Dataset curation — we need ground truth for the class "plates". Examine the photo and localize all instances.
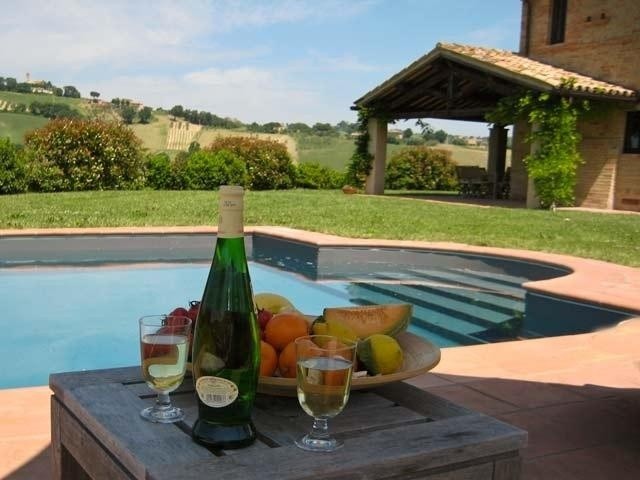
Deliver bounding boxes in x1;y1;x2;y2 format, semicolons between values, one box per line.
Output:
184;329;441;398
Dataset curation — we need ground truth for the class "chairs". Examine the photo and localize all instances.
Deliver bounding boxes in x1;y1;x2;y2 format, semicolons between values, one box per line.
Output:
454;165;512;201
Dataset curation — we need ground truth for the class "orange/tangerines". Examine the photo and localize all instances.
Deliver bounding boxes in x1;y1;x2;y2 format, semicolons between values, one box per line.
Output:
259;314;319;377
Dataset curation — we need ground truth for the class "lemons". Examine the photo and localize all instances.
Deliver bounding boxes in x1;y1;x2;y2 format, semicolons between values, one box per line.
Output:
362;333;404;374
312;320;359;350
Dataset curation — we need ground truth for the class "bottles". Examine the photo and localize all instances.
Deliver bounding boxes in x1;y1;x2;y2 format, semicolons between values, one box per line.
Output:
189;186;261;449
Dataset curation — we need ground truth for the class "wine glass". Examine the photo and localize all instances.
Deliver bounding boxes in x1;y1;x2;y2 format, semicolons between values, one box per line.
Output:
293;335;357;453
139;315;194;424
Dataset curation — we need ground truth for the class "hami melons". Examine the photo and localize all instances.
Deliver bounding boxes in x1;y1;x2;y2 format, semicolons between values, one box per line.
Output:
323;304;413;340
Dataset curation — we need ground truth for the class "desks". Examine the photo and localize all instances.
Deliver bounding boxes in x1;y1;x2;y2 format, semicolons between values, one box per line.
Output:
47;364;530;480
